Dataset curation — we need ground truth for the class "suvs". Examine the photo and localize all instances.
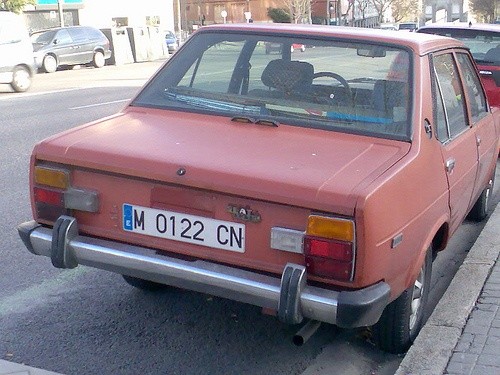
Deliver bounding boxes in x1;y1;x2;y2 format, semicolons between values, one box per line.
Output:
29;25;112;73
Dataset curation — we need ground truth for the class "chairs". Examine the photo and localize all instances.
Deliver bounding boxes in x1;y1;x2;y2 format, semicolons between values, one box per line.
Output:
247;59;314;101
370;78;409;113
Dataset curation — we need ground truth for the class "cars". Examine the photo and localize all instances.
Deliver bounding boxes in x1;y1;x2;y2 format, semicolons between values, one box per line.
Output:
164;30;176;54
1;11;35;93
387;22;500;107
16;22;500;355
382;23;416;32
